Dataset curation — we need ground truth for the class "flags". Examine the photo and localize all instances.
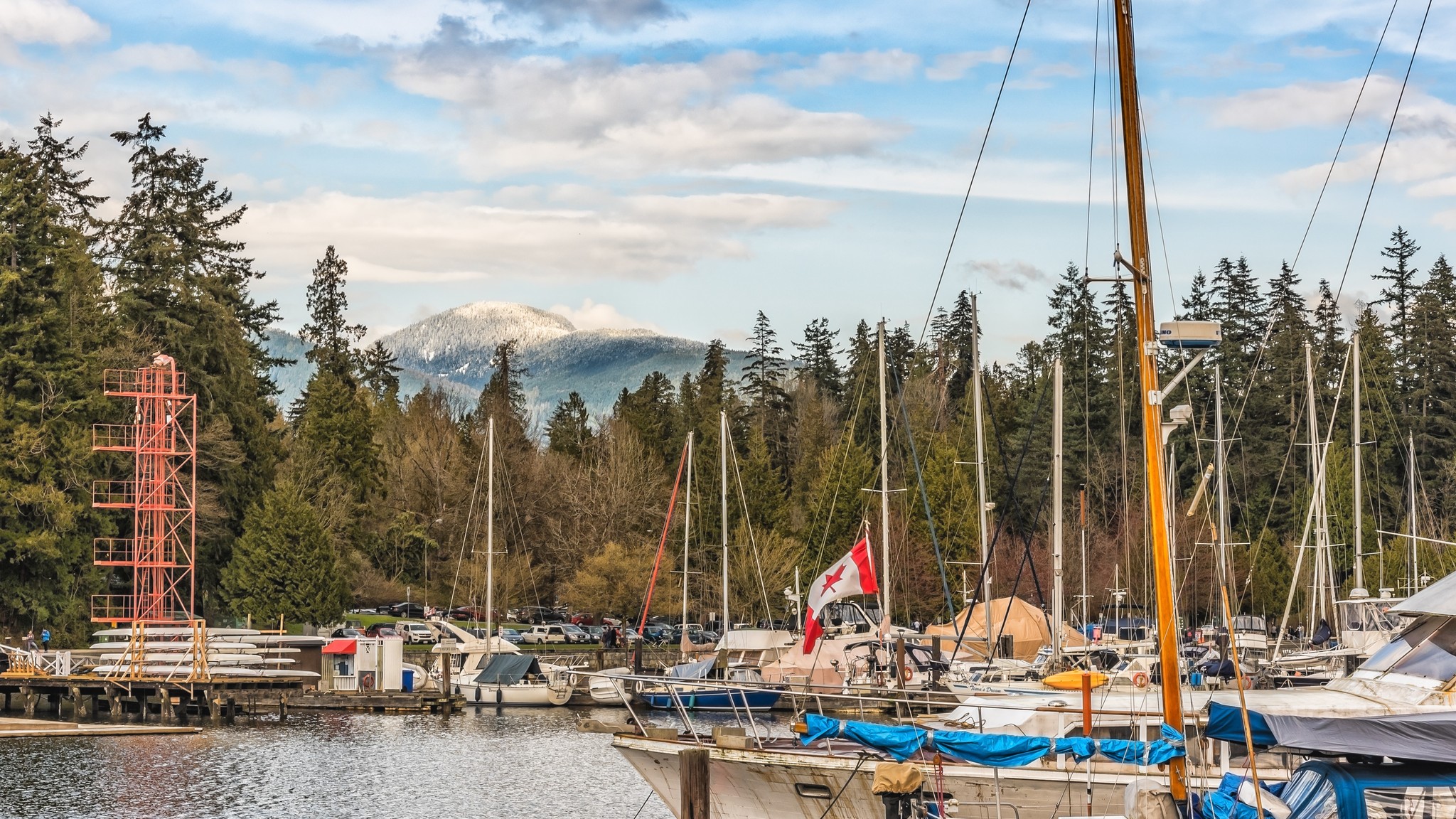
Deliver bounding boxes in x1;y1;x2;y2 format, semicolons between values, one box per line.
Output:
426;608;435;615
803;527;879;657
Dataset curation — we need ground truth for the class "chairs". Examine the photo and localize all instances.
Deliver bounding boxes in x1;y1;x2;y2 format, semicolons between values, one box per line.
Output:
688;631;692;634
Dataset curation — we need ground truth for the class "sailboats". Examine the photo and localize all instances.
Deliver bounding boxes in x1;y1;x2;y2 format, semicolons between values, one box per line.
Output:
402;0;1456;819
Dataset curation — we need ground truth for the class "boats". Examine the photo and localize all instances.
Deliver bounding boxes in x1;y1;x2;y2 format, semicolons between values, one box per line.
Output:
89;627;321;677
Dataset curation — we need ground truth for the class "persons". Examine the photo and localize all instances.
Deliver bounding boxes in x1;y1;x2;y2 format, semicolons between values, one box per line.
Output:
40;627;50;653
27;631;40;653
601;626;623;649
1271;623;1304;641
911;620;923;634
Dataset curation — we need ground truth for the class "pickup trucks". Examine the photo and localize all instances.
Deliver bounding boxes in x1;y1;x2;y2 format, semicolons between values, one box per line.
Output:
520;625;566;645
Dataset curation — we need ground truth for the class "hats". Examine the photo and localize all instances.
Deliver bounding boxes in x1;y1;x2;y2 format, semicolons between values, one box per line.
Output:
617;627;620;630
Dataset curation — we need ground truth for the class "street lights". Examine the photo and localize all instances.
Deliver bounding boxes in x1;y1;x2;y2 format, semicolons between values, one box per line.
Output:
425;519;442;621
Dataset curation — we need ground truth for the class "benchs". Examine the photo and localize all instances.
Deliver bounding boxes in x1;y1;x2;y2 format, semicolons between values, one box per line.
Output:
599;640;632;648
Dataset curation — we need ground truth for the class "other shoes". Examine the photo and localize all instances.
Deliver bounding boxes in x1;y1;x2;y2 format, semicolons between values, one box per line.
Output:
44;651;48;653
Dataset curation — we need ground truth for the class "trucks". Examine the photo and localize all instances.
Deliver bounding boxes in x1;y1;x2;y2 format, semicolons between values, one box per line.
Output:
394;620;436;645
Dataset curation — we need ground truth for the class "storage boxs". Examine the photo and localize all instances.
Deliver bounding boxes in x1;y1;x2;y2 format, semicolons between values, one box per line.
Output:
711;726;746;742
717;735;754;749
644;728;679;742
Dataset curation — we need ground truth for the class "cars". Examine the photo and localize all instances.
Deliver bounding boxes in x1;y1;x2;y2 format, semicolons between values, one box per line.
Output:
368;628;404;645
1200;625;1218;638
570;613;613;627
493;628;524;644
603;613;630;629
670;628;703;645
699;631;721;644
451;606;502;623
614;628;644;644
388;602;435;619
331;628;366;638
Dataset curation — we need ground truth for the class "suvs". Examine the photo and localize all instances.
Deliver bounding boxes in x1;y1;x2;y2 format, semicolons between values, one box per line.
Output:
516;606;565;625
757;618;796;630
551;622;591;644
578;625;606;644
635;626;674;645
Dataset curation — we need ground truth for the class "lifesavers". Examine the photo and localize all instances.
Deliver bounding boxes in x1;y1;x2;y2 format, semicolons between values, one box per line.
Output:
437;674;442;678
363;675;374;688
1133;672;1148;688
1241;676;1251;690
636;681;642;694
570;673;577;686
904;667;913;681
